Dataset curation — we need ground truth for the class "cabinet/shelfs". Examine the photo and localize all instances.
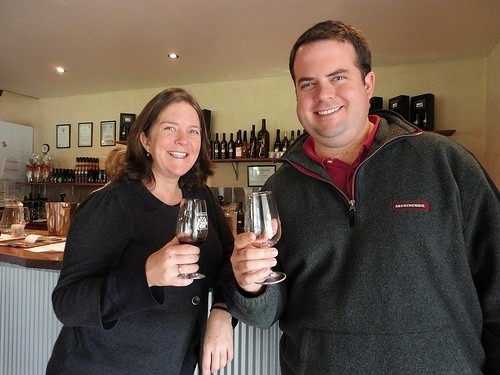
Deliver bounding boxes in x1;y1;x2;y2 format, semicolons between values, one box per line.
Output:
16;181;105;231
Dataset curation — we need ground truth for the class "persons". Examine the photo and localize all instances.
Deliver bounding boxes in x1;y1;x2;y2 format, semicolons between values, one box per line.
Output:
46;87;238;375
227;21;500;375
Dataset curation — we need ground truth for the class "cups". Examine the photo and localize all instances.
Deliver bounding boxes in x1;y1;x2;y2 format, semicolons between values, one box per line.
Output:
99;170;110;183
11;224;24;238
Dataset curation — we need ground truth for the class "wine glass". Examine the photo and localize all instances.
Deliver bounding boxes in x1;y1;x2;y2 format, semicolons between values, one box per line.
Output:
175;198;208;280
243;191;287;285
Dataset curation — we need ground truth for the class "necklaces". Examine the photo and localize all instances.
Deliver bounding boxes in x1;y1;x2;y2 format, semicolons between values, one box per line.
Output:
159;188;180;205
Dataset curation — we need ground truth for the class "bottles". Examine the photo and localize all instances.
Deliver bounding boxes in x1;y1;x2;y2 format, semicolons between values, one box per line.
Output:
50;156;100;183
208;119;307;160
237;202;244;234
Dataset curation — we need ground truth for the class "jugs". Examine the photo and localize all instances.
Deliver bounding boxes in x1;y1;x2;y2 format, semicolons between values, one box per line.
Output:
0;197;30;234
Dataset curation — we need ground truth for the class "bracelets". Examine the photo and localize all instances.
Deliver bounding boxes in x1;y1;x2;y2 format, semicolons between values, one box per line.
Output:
210;305;227;311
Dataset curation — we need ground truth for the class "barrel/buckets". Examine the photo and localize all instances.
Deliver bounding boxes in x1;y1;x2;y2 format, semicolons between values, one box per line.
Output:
45;201;77;237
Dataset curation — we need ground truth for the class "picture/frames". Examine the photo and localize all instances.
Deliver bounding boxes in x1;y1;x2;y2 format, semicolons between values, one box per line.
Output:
78;122;93;147
56;124;71;148
100;121;116;146
247;165;277;188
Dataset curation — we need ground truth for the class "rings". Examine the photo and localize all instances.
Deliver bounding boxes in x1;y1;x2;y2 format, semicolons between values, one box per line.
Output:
178;265;182;274
